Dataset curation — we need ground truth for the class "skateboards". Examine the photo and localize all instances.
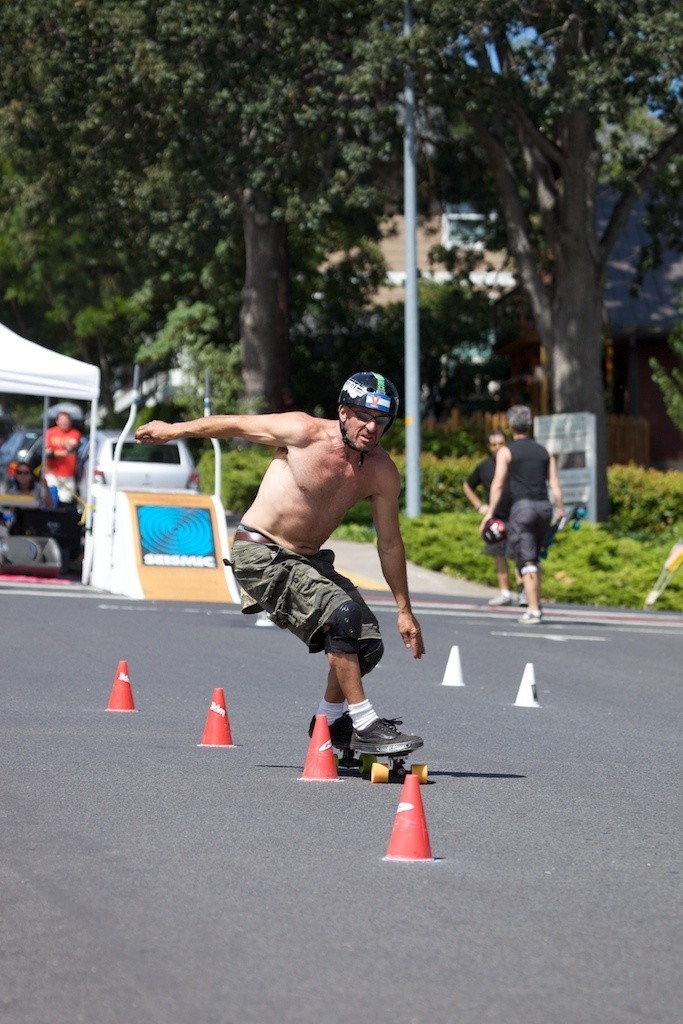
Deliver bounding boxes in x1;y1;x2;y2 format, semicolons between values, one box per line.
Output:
537;501;588;561
328;735;429;784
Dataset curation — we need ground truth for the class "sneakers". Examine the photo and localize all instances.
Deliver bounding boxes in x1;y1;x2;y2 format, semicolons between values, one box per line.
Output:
307;710;353;751
350;716;424;753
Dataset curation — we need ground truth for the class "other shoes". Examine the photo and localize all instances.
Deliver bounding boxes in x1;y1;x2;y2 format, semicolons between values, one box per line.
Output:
488;592;512;606
518;611;543;624
518;589;528;607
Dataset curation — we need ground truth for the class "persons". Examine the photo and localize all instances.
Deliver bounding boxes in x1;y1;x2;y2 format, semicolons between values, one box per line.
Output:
478;406;564;623
7;462;53;536
463;429;529;606
136;371;426;753
48;411;82;505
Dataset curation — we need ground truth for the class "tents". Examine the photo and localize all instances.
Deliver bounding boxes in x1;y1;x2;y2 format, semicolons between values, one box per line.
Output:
1;323;101;582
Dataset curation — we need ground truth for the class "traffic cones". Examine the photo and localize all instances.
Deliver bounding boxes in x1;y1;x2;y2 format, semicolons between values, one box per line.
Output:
512;663;540;708
198;688;237;747
439;646;467;686
297;713;342;781
104;660;138;713
381;775;435;861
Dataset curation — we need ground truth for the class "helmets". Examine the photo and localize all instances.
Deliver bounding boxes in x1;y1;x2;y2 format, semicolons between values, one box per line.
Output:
483;518;507;544
338;371;399;417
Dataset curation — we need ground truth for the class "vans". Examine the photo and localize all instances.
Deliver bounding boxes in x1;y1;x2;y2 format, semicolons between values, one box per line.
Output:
78;430;198;524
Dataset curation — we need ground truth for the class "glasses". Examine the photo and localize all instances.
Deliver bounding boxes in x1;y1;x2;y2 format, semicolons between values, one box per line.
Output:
344;404;389;425
16;470;31;474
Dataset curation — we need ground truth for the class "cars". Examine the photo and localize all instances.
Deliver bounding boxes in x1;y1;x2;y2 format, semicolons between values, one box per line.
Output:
0;431;43;493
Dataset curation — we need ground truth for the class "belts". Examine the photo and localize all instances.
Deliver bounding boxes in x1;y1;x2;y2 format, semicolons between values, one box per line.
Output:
235;532;274;544
